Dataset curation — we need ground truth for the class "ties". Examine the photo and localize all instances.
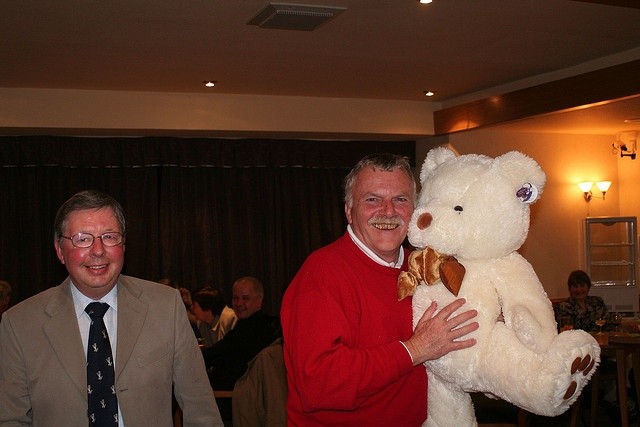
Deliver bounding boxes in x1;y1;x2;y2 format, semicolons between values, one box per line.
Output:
84;302;119;427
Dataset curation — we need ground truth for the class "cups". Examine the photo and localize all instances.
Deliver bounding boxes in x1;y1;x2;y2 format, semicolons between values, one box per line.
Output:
559;316;574;332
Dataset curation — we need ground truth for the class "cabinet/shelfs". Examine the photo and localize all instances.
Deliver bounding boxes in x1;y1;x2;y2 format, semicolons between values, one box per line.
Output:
580;216;639;317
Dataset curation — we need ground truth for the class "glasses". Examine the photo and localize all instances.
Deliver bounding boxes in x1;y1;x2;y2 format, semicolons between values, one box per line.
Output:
62;233;125;247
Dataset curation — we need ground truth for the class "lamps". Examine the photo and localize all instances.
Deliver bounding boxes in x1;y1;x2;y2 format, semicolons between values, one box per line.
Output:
579;180;593;202
597;180;612;200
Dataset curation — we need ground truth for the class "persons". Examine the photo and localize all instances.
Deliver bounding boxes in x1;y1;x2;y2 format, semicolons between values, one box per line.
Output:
0;189;226;426
0;281;12;313
279;153;480;427
556;270;614;332
189;285;238;348
154;278;194;312
202;275;283;421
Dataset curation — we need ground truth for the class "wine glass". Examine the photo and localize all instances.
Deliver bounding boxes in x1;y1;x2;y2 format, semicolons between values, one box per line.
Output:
594;312;606;335
610;312;622;335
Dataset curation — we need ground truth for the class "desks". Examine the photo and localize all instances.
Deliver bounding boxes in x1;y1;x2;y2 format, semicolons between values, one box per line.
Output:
570;330;640;426
